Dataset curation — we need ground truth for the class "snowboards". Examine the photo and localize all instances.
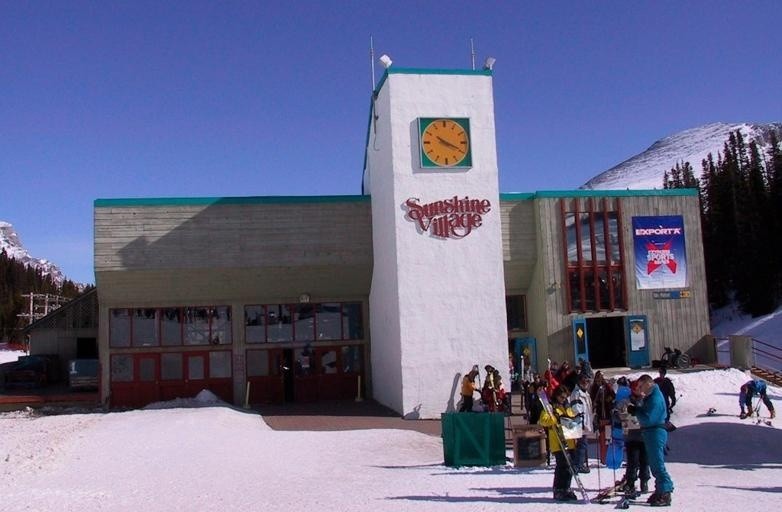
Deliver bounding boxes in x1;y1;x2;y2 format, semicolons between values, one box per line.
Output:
605;385;631;469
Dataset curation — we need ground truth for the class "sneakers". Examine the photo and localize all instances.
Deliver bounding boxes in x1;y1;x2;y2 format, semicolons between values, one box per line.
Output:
553;489;578;502
641;481;648;492
618;479;635;492
646;492;672;507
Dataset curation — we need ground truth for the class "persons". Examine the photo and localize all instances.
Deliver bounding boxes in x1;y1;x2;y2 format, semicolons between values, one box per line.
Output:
459;350;679;507
738;378;775;419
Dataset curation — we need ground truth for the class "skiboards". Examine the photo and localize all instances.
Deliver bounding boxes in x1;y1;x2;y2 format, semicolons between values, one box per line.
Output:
520;355;525;409
595;476;626;503
488;372;499;414
537;386;590;505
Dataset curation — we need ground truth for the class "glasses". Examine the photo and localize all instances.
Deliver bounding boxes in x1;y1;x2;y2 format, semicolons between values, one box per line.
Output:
560;396;569;400
637;379;650;389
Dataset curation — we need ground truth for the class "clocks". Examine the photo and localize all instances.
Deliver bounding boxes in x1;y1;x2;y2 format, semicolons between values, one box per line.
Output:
419;118;473;169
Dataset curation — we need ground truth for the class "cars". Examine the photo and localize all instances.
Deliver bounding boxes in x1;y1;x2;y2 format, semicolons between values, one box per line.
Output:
0;355;68;390
68;359;100;393
